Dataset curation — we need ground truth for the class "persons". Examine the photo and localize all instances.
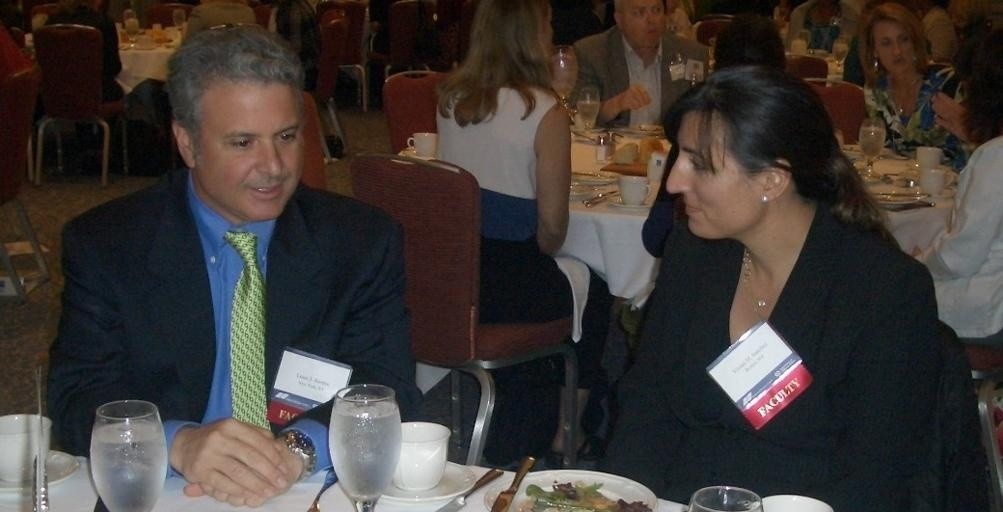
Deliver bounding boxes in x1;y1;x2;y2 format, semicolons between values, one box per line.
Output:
912;7;1003;350
565;0;694;132
772;2;1003;171
186;3;256;38
601;64;942;512
434;2;612;465
2;2;122;173
642;10;787;258
45;21;413;504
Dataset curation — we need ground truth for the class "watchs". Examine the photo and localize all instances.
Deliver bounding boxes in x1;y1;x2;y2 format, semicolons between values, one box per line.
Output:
282;427;317;486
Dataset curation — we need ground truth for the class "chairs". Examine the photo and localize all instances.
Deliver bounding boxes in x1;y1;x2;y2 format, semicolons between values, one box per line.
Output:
961;339;1003;512
351;154;578;469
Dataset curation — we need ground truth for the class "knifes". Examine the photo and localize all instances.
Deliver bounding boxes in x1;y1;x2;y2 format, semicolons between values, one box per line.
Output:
489;456;536;512
435;469;503;512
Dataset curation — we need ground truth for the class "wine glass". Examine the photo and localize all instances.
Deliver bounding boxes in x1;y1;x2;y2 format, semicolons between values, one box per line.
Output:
550;46;579;117
859;117;886;176
689;485;764;512
576;88;600;143
122;8;186;37
790;30;849;75
328;384;403;511
88;399;168;511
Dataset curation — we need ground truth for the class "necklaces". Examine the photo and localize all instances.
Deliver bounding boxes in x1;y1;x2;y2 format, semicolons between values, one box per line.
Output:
743;248;779;325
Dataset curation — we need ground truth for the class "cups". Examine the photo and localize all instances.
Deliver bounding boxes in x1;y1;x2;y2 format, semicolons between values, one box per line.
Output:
393;421;451;492
406;133;436;159
0;413;54;482
762;494;834;512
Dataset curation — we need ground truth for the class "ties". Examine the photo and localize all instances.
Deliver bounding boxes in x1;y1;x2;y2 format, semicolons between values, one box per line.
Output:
224;228;269;430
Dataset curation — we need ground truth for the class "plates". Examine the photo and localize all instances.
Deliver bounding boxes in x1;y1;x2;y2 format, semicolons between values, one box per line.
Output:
0;450;80;491
317;465;532;512
483;469;658;512
381;460;476;501
134;43;160;50
570;123;959;210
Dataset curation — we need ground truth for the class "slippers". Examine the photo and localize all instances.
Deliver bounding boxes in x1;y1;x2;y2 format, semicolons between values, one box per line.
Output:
545;440;602;469
